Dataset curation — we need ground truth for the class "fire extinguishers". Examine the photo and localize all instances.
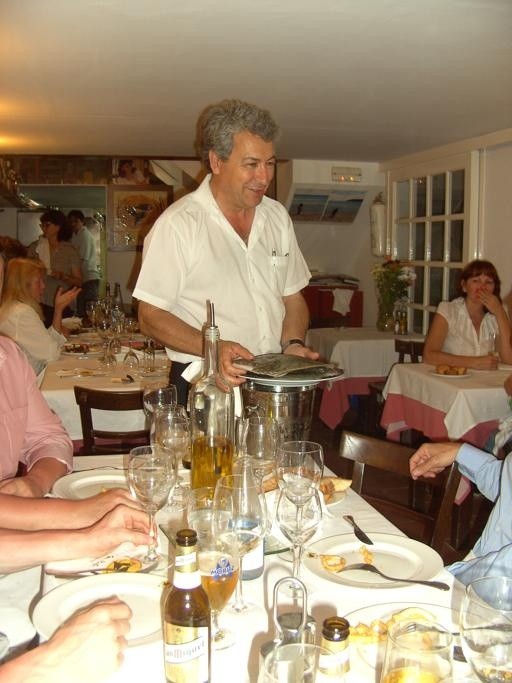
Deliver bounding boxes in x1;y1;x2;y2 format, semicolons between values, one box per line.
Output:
369;191;387;257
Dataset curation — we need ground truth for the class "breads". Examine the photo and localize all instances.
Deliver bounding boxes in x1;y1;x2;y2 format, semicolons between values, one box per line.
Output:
436;364;466;375
258;465;353;504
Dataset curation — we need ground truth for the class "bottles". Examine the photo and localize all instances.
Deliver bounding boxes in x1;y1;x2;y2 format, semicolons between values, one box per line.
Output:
189;300;237;502
102;281;113;305
113;283;124;306
394;309;408;333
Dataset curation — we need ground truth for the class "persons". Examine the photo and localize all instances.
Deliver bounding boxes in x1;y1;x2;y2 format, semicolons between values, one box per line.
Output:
410;441;511;611
68;209;102;318
1;338;75;500
1;256;82;388
1;487;142;530
131;98;320;434
0;506;157;678
422;259;511;370
25;208;83;329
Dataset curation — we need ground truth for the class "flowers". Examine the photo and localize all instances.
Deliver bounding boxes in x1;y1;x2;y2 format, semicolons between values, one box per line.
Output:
369;252;418;314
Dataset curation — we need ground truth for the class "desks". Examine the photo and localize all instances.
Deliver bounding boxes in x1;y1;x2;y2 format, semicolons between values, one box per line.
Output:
300;272;363;325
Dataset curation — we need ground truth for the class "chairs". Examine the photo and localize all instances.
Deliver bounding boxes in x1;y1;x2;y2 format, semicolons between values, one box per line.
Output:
438;451;501;565
363;336;423;440
340;430;457;563
75;384;166;455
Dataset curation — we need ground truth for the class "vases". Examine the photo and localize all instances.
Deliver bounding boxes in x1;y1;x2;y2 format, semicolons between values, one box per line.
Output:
377;299;397;330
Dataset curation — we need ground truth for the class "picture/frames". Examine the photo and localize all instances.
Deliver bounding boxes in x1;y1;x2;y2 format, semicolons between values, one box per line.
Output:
108;185;174;251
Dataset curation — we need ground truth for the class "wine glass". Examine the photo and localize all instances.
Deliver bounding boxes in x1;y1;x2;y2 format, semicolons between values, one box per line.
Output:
128;383;326;613
79;299;158;374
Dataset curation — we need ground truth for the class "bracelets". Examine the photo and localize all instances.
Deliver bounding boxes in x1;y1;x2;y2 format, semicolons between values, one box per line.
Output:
281;338;305;352
64;273;69;282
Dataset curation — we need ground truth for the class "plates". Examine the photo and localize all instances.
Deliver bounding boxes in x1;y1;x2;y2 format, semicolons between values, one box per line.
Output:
237;369;344;387
29;467;511;681
432;372;472;378
61;349;101;355
